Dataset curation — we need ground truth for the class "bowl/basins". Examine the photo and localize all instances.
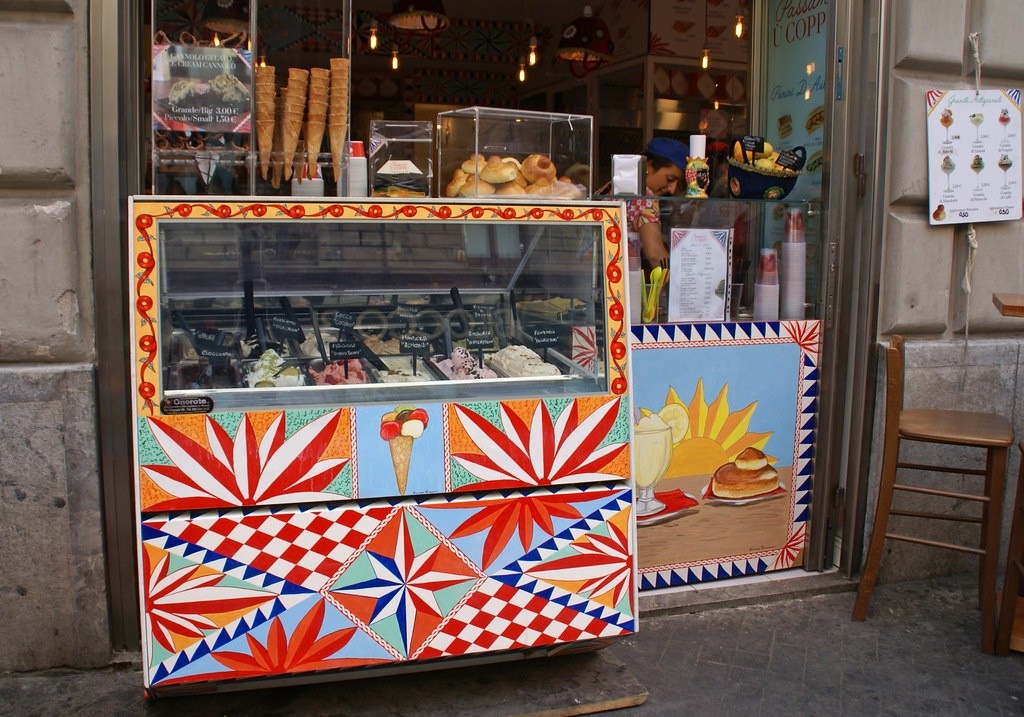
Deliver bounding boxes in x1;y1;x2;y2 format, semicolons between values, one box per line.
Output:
162;321;562;391
290;164;324;196
336;141;368;197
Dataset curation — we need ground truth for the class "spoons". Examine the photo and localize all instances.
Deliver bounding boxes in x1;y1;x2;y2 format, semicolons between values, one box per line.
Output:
641;267;670;323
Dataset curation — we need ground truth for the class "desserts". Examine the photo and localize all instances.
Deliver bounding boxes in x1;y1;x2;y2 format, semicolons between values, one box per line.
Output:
167;74;248;106
941;108;1011;170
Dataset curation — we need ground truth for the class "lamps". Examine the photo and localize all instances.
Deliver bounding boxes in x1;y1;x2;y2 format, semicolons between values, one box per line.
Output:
201;0;252;35
384;0;451;36
555;1;614;77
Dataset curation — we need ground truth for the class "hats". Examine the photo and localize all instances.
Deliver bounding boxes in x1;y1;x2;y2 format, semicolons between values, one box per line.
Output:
648;138;690;171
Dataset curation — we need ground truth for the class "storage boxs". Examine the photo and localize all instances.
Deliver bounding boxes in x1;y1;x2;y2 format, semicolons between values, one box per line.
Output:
539;0;750;77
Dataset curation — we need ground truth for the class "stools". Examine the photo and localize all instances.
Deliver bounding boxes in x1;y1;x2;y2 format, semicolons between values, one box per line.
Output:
852;331;1015;654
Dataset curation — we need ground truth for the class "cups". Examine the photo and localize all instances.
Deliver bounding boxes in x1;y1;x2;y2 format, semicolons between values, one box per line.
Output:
640;284;661;324
753;208;807;322
627;231;642;324
730;283;745;320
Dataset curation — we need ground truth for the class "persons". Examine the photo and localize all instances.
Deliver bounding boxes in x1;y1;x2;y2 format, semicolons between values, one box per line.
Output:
600;137;688;274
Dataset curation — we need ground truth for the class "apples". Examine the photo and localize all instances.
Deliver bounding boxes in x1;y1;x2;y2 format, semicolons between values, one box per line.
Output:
734;140;792;172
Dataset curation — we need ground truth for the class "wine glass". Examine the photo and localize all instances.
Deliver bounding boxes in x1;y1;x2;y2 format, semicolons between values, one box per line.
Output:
940;117;1013;192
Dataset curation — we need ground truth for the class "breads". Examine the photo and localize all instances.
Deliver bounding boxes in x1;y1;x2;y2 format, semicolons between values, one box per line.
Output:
446;153;580;199
